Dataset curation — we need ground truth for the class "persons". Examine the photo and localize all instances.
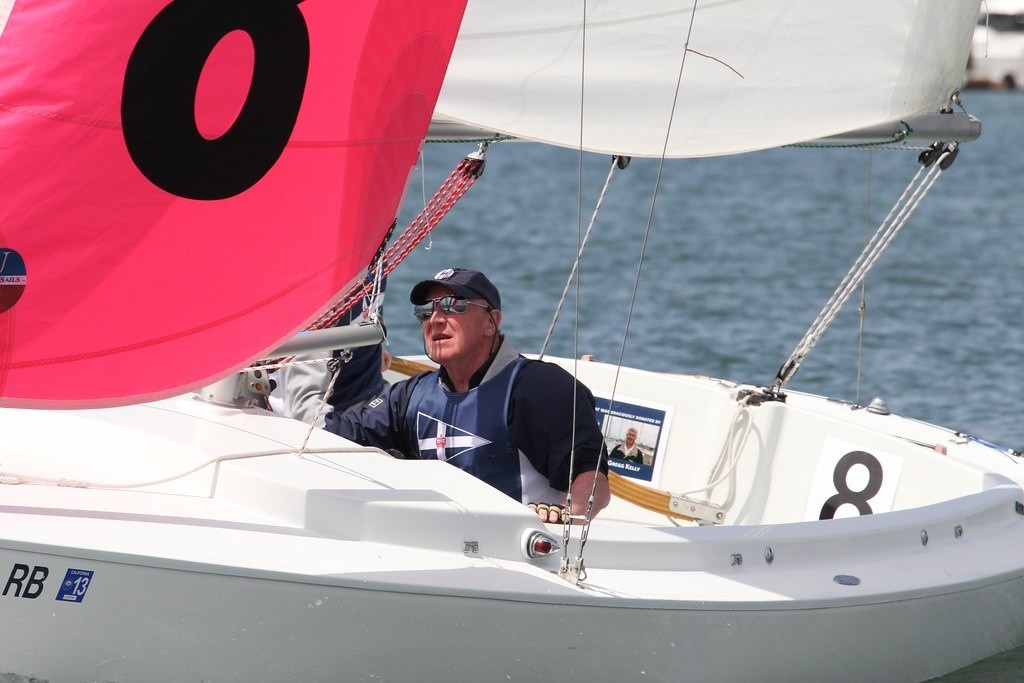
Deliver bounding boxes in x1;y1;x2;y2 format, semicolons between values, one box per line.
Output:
609;427;644;464
323;268;610;526
265;244;392;429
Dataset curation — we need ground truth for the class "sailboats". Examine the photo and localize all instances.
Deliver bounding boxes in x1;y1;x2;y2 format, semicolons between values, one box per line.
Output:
0;0;1023;683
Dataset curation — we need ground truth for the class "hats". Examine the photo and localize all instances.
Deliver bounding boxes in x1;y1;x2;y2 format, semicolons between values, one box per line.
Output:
410;268;502;313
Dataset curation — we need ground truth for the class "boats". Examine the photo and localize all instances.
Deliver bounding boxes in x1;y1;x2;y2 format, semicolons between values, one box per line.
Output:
967;0;1024;93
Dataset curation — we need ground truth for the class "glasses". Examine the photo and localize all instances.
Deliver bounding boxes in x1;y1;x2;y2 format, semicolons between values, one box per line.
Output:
413;294;492;321
